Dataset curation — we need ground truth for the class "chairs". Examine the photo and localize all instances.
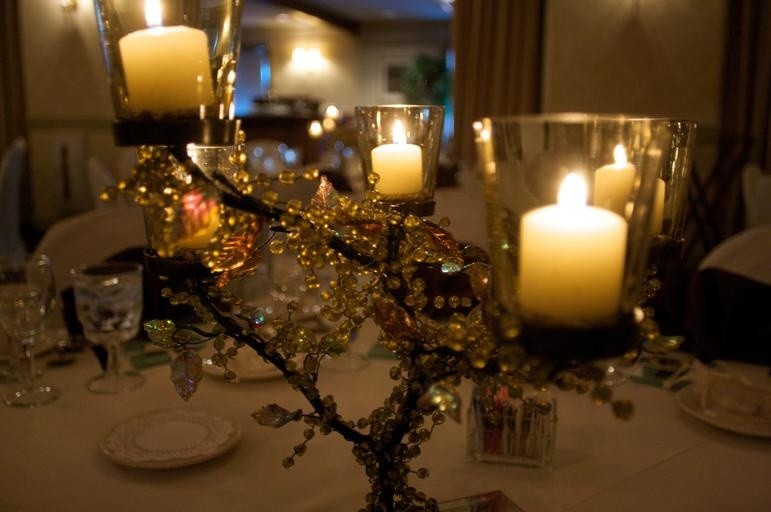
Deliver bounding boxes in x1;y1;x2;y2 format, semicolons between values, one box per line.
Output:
0;135;29;275
21;206;227;337
688;224;771;360
725;166;771;236
424;185;494;252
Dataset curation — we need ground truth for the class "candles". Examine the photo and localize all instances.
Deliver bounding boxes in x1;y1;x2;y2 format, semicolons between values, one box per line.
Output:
520;170;628;320
595;143;637;213
118;1;216;115
370;119;423;194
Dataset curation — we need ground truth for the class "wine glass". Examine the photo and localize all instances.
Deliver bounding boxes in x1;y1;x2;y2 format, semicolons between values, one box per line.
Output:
68;261;148;393
1;252;63;410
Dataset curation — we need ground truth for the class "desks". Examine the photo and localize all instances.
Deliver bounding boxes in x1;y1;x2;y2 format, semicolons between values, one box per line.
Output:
1;299;771;510
236;114;356;175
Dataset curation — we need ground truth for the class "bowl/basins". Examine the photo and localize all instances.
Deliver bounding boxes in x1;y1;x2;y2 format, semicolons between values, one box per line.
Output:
463;393;560;470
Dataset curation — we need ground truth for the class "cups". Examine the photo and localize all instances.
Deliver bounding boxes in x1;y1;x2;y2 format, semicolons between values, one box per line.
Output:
92;0;244;116
352;99;443;203
487;109;698;361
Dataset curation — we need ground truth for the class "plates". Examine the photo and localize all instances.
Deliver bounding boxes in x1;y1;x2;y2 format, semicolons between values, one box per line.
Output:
199;341;294;382
103;403;244;470
676;380;770;437
